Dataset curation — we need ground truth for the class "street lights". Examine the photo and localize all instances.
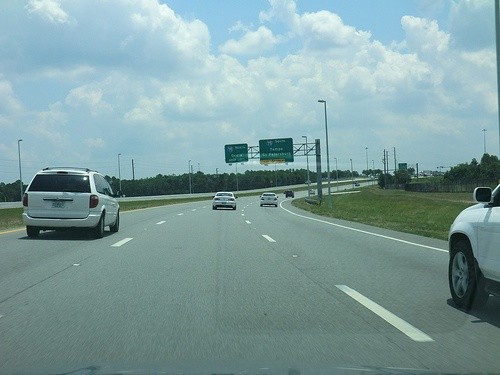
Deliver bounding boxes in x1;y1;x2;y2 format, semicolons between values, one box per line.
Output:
349;158;354;189
333;157;339;191
259;192;279;207
372;159;374;185
302;136;310;197
117;152;122;190
188;159;192;194
318;99;331;208
17;138;24;199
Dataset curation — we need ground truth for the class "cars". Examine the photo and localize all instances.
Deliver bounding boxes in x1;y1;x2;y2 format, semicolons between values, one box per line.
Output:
309;189;315;195
285;190;294;198
447;182;500;311
305;180;311;185
354;182;361;186
213;191;238;210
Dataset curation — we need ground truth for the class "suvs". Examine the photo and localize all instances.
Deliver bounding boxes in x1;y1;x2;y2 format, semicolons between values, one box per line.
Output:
22;166;121;238
